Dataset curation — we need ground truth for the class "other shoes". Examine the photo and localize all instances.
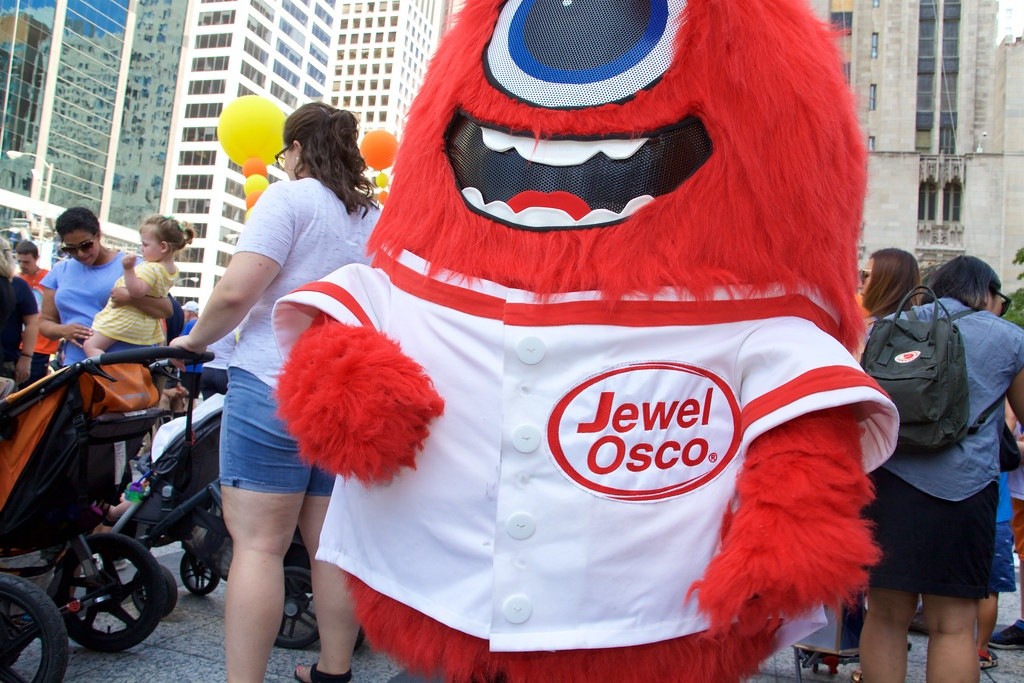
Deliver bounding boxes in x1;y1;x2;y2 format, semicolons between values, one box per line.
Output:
978;650;998;668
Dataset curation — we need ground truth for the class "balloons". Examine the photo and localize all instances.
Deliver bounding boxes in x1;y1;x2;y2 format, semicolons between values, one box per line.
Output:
376;174;389;205
361;130;399;172
217;94;287;168
242;157;269;221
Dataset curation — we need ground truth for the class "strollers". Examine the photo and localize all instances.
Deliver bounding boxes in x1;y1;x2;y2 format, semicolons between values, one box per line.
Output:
0;345;365;683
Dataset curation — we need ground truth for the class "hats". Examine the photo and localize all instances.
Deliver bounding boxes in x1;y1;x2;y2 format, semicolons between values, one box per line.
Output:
181;301;199;313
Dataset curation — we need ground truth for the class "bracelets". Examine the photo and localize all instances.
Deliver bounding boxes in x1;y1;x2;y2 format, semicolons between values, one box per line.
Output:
19;353;33;359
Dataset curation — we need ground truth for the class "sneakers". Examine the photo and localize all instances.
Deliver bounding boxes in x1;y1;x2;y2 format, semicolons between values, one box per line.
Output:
988;619;1024;650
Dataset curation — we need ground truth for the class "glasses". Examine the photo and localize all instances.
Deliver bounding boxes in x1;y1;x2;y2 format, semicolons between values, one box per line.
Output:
861;268;872;284
60;234;95;255
989;287;1012;317
274;146;294;168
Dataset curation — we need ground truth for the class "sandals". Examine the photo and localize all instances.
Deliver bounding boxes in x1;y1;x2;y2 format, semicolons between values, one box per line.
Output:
294;663;352;683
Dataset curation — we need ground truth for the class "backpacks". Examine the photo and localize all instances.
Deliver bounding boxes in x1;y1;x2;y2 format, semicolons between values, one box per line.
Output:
860;285;1003;452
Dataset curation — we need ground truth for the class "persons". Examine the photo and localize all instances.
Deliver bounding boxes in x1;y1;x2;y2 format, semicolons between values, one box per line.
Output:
170;100;382;681
89;299;239;522
841;249;921;663
907;392;1023;669
83;214;193;370
0;234;64;393
20;539;82;622
851;254;1023;683
38;207;175;581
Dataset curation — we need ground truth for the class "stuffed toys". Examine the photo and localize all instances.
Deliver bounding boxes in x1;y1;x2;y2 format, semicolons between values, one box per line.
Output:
271;0;899;682
